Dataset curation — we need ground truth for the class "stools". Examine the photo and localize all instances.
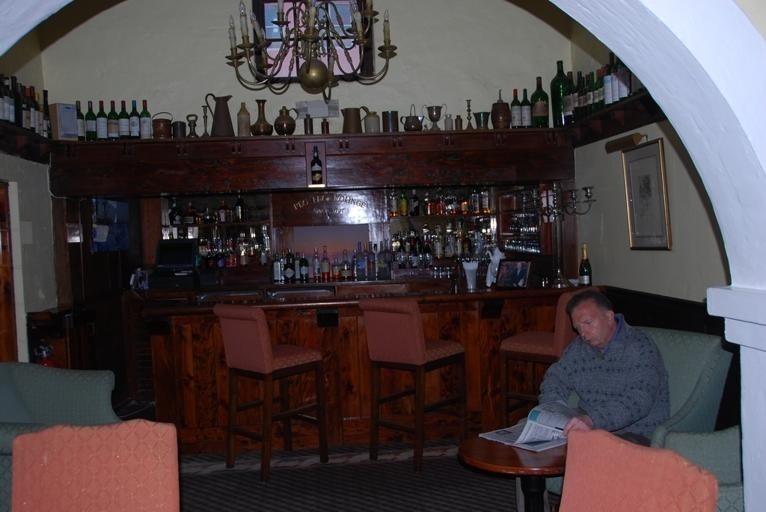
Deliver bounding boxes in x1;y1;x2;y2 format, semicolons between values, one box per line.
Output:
358;298;467;474
214;303;330;482
500;290;577;429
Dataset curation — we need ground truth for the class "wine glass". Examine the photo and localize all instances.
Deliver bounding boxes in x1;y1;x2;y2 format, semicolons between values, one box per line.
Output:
426;106;442;132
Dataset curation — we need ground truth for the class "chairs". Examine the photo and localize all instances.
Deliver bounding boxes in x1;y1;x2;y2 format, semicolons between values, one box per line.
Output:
11;418;180;512
2;360;122;512
558;428;718;512
516;325;733;511
664;425;744;511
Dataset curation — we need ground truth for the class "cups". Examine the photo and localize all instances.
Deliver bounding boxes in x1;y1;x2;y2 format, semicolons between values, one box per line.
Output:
382;110;399;132
172;121;186;137
473;111;490;129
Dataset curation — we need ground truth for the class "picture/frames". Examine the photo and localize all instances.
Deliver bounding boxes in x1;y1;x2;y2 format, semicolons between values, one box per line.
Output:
620;138;672;250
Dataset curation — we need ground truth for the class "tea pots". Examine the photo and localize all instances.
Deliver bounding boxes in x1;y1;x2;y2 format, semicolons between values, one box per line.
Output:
340;104;370;133
274;105;299;135
400;104;425;131
203;93;236;136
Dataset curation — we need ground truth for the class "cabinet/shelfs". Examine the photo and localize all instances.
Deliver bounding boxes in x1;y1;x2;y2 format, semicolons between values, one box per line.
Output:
27;304;95;371
49;128;578;291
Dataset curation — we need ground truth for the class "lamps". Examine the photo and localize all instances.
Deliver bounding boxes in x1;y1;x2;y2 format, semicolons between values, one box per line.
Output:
225;1;398;105
605;133;648;154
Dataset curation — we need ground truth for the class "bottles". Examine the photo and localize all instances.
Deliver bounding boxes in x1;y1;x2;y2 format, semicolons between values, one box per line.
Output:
168;188;247;225
321;119;330;134
386;186;489;218
75;99;151;141
235;102;251;138
392;254;492;279
365;111;380;134
502;221;540;254
0;73;52;138
303;114;313;135
390;220;491;254
511;52;641;130
310;145;322;182
200;224;271;269
274;239;391;282
578;244;593;286
445;114;463;131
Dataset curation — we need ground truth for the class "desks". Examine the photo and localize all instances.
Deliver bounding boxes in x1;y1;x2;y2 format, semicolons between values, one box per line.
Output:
459;424;616;512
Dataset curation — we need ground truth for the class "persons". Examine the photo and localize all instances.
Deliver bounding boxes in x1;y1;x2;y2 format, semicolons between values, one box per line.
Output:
538;290;670;512
500;262;526;287
